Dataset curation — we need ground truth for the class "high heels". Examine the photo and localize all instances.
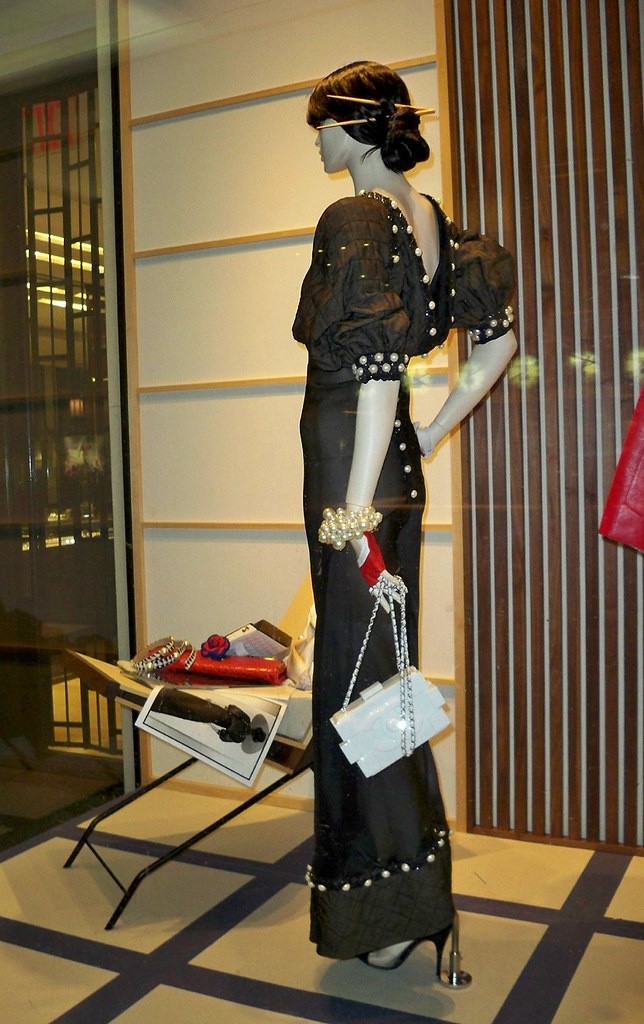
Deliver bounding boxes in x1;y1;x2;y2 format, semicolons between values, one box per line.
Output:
357;920;452;979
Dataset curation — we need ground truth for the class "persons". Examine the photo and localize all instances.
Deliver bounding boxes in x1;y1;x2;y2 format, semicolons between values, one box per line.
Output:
285;57;522;980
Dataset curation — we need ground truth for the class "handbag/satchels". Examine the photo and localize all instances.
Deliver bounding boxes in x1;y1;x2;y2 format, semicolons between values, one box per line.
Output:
330;576;450;779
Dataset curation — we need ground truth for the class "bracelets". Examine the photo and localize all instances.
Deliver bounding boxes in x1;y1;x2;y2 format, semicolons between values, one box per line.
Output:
316;506;383;551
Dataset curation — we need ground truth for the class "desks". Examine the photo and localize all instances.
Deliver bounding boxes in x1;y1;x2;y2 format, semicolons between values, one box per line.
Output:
55;647;313;932
